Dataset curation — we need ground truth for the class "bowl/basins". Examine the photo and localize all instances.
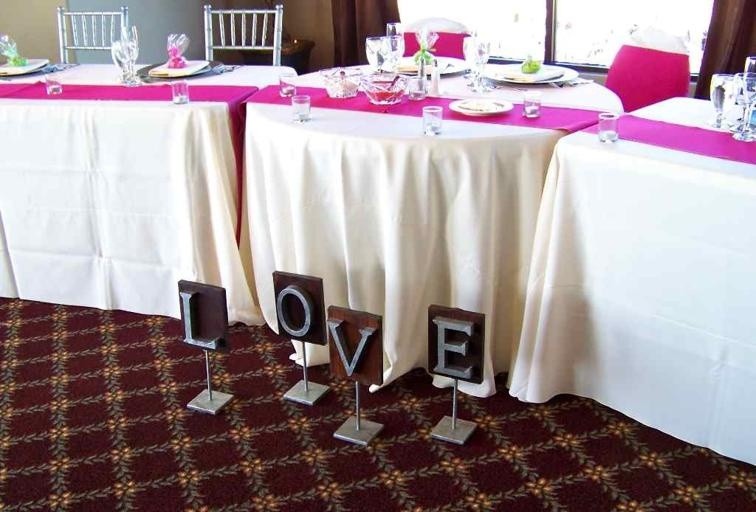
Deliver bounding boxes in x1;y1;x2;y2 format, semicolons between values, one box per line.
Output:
358;74;408;105
318;66;361;100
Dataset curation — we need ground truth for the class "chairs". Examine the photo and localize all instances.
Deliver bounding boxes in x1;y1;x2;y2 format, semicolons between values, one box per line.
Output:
203;5;283;66
57;5;129;65
604;45;691;113
404;33;472;58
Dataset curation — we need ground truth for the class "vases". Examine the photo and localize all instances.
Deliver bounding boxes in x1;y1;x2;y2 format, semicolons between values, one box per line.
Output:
240;39;315;74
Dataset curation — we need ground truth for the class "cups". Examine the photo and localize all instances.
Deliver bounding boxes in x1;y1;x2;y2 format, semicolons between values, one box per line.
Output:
365;35;405;70
278;73;297;98
522;91;541;118
408;75;425;101
290;94;310;122
170;78;190;105
44;72;61;95
598;111;619;145
421;105;443;136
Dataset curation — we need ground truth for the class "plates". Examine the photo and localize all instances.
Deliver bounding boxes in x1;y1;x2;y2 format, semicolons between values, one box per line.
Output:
0;58;49;76
147;60;211;77
448;97;513;116
482;62;580;84
395;54;464;75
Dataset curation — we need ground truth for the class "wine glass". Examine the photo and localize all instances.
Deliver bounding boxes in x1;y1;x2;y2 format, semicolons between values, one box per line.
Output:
707;55;756;143
463;36;493;94
384;23;405;36
109;26;142;89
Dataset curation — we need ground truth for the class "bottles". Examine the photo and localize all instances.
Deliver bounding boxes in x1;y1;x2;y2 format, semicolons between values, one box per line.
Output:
430;60;439;96
